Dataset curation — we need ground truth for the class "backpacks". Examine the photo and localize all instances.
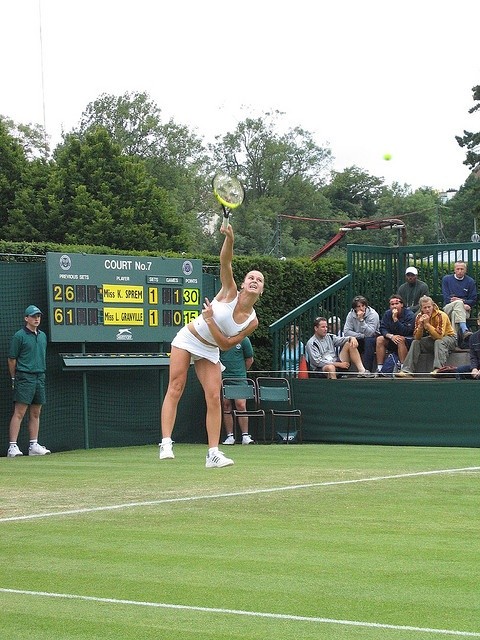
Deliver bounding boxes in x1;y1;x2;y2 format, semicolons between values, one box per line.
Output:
379;350;400;378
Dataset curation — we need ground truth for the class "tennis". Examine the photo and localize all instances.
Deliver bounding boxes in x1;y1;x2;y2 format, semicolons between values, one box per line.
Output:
384;153;392;160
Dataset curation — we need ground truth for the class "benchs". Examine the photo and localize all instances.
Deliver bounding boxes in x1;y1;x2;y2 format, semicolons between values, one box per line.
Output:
372;350;472;376
459;316;479;347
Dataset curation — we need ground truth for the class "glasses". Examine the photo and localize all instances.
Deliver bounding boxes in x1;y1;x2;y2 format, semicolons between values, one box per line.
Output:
356;297;365;301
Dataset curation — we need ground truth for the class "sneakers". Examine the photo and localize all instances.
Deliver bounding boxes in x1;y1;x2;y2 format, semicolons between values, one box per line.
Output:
205;450;235;468
242;434;254;445
28;443;51;456
357;370;376;378
396;371;413;378
159;440;175;461
462;330;473;341
223;436;235;445
430;369;439;377
7;444;23;458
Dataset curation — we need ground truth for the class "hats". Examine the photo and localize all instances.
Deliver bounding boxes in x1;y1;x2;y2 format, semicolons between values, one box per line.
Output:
25;304;42;317
405;266;418;275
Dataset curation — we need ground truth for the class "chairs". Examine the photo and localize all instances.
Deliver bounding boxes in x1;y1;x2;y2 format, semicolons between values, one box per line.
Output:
256;377;303;446
220;376;268;446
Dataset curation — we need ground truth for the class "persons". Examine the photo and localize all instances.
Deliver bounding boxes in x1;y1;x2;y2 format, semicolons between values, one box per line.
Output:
397;267;428;316
219;338;255;445
341;295;381;378
327;316;343;356
374;294;416;378
281;325;309;377
443;261;477;348
455;312;480;380
7;305;52;456
395;294;457;378
158;222;265;467
306;317;375;379
